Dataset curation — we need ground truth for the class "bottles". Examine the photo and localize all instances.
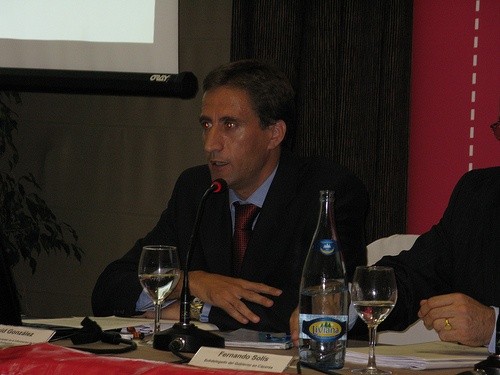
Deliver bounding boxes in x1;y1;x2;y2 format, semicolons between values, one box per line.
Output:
298;191;351;369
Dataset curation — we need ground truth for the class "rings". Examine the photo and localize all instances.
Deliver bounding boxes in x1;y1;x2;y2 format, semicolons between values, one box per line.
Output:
445;318;450;327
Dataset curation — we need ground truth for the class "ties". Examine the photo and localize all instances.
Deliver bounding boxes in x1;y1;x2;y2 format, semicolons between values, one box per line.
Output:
232;201;261;266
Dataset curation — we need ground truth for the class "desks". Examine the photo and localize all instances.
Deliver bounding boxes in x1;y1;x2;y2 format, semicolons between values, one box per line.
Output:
0;311;500;375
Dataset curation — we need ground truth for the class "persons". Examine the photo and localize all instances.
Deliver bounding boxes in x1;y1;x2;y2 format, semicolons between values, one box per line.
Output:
291;164;500;355
90;63;337;333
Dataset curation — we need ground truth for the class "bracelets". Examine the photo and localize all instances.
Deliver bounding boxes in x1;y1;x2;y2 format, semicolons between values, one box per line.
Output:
189;301;204;320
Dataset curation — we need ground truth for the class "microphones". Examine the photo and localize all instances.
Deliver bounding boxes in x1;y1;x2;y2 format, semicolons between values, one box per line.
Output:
152;178;230;353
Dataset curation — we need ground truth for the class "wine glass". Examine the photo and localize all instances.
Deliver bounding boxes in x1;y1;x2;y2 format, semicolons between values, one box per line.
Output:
137;242;182;347
348;266;396;375
